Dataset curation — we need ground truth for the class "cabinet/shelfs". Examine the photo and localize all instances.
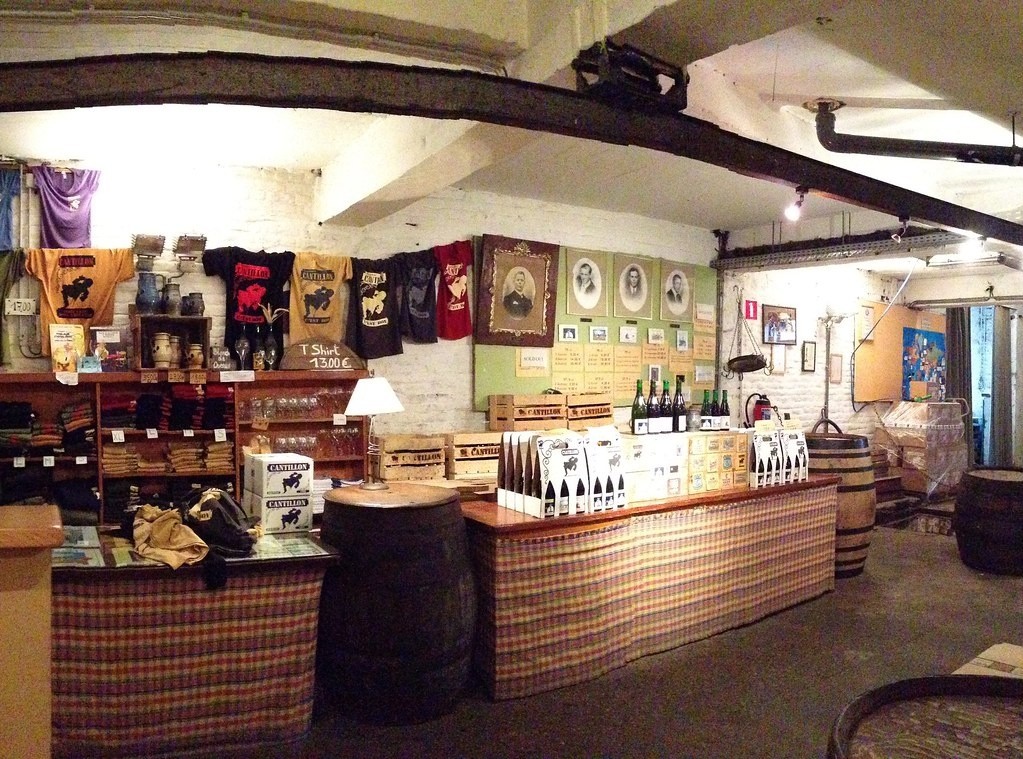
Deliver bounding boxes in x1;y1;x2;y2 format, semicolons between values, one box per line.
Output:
0;369;370;526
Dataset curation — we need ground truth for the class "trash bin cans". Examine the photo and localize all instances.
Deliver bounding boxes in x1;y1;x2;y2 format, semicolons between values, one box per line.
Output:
973;417;984;465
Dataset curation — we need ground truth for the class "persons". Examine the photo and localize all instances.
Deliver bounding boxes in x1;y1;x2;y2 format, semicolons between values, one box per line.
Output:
667;274;682;303
626;268;642;299
579;264;596;294
503;271;532;316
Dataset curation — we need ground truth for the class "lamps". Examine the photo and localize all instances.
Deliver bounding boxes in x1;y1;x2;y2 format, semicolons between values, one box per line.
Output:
171;232;208;272
889;215;913;245
783;185;809;220
130;231;166;270
964;236;987;260
343;377;406;490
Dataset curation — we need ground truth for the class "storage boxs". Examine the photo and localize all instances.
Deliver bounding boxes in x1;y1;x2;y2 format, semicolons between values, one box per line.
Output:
241;489;313;534
243;453;314;497
369;433;447;481
443;431;502;479
567;393;615;431
129;316;213;370
874;380;969;495
488;391;568;432
620;430;747;503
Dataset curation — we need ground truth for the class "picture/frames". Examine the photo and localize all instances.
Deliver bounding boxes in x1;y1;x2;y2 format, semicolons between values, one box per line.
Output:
474;232;561;348
660;259;697;323
677;330;688;351
613;253;653;321
649;363;661;386
761;303;797;345
803;340;817;373
828;352;844;384
770;344;787;375
558;323;579;342
647;328;664;344
619;326;637;344
589;325;609;344
565;248;609;317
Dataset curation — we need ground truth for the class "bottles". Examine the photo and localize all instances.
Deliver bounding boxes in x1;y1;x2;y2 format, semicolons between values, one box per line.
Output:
699;390;731;431
659;380;674;433
747;427;807;489
631;379;650;434
674;376;687;433
646;380;661;434
499;431;626;520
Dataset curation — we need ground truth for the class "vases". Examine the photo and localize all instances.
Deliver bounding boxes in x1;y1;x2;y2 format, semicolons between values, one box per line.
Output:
135;272;206;316
150;332;203;370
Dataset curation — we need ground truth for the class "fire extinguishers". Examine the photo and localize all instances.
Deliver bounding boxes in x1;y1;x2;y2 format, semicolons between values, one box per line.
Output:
745;393;771;428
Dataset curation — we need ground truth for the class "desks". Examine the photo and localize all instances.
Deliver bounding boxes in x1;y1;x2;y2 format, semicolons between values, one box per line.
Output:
51;531;348;759
460;472;842;702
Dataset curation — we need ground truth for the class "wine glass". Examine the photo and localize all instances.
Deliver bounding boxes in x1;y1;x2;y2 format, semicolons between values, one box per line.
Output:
238;384;363;462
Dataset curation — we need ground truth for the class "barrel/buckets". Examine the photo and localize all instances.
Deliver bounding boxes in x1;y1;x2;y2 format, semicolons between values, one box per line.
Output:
800;432;877;578
315;479;479;723
952;464;1023;574
